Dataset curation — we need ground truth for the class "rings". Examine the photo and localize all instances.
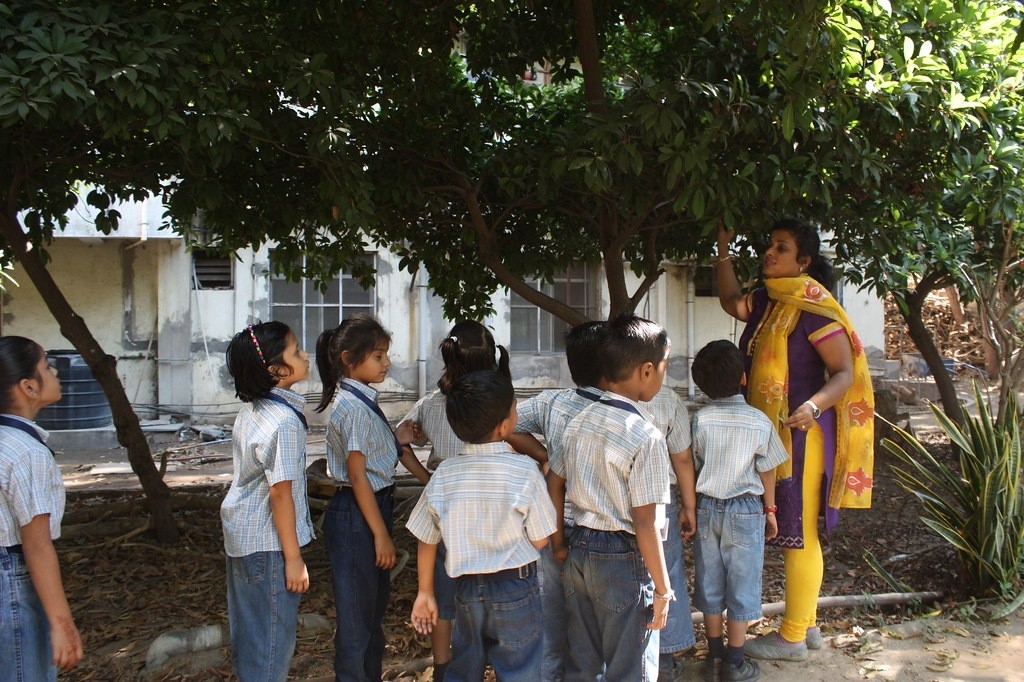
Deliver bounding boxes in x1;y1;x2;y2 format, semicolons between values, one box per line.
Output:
802;425;805;430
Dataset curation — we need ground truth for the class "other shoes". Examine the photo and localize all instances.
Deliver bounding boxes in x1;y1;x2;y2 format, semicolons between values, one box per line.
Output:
657;659;684;682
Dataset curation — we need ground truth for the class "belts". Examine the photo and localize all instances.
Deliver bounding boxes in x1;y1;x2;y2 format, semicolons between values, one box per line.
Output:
337;482;397;497
456;560;537;582
6;544;24;554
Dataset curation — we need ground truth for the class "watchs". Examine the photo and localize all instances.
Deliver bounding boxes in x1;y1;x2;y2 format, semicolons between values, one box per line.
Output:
804;401;821;419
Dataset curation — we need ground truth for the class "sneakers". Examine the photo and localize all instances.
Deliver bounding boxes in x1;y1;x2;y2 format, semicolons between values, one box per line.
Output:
703;652;726;682
743;631;808;661
806;626;821;650
720;656;762;682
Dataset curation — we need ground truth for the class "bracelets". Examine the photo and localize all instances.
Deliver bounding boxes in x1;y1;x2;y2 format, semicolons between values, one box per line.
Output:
651;590;675;602
764;505;777;512
716;256;731;265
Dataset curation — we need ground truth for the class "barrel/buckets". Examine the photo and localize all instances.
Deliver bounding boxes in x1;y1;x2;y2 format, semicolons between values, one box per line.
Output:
34;349;113;431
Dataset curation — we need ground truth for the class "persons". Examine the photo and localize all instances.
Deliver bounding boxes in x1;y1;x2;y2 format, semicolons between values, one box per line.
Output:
718;217;875;661
0;336;82;682
692;340;789;682
638;386;696;682
316;316;419;682
548;316;670;682
395;320;510;682
220;320;316;682
406;368;557;682
506;321;606;682
